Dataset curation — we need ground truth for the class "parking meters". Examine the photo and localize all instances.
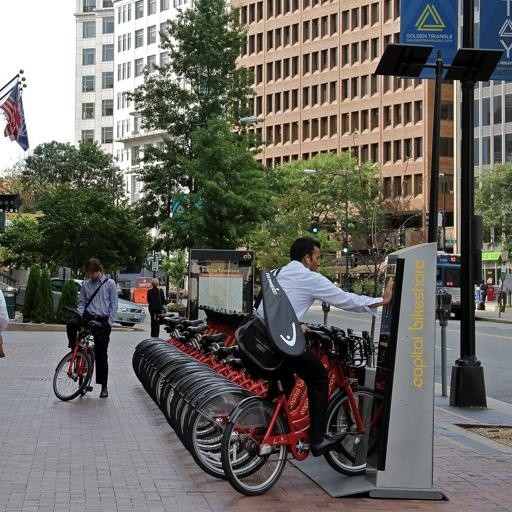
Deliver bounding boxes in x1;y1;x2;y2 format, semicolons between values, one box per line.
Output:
435;288;452;396
322;301;330;326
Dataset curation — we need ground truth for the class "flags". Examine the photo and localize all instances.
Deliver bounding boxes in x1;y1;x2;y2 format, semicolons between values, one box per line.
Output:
1;77;29;152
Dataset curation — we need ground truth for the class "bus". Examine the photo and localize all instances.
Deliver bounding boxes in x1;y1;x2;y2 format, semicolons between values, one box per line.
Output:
436;251;462;320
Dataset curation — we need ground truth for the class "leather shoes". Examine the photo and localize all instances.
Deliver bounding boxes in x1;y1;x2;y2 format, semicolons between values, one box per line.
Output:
99;387;109;398
310;430;349;457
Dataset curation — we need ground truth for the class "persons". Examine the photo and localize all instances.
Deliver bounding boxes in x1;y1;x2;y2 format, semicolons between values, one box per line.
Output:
147;278;165;337
480;280;488;303
251;238;394;457
0;289;10;357
499;276;507;312
67;257;118;398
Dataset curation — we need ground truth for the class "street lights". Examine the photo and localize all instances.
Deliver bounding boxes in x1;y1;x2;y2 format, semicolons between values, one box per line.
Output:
182;116;264;307
303;169;349;275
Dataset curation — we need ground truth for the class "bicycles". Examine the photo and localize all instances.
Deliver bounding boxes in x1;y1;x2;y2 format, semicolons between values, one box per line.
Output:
52;306;108;401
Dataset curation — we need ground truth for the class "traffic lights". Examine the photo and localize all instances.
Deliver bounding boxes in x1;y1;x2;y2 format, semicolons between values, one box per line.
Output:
310;213;320;235
343;238;348;255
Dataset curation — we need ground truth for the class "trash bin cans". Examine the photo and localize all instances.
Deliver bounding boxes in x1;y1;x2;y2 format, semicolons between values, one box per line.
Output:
2;287;17;319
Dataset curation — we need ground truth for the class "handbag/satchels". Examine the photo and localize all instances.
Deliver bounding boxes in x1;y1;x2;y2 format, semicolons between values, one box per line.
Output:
234;312;286;381
160;304;168;314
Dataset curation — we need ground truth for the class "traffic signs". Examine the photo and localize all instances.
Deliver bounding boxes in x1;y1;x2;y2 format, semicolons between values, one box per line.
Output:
399;0;458;79
152;259;159;272
479;0;512;80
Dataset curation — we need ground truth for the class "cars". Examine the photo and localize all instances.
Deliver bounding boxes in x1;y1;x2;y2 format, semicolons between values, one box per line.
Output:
15;277;146;326
